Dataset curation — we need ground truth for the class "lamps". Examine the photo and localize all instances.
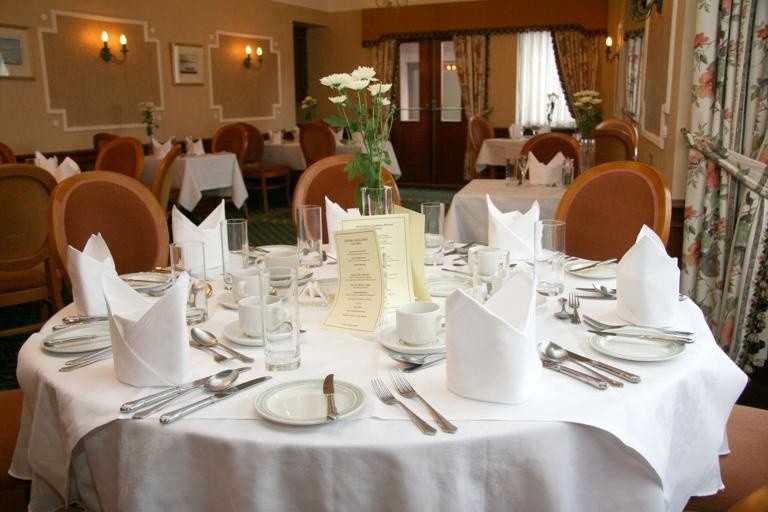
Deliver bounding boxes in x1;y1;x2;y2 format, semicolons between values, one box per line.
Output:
600;35;619;62
242;46;265;71
98;29;131;64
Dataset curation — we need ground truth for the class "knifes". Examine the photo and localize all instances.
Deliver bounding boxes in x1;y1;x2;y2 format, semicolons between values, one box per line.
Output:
403;357;446;372
542;359;609;391
158;375;273;425
120;366;252;413
443;239;475;256
321;372;340;423
588;328;694;346
551;342;641;384
568;258;618;272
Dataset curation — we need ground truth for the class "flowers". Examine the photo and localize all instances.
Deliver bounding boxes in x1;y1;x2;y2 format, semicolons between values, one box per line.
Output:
317;67;399;208
574;86;603;140
140;99;158;135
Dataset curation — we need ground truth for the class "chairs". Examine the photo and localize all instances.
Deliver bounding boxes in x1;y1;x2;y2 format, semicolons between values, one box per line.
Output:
471;114;673;266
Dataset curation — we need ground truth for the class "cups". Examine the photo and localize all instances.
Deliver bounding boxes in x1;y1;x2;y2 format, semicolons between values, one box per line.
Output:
533;128;551;135
297;205;324;268
261;267;301;371
472;248;510;303
237;295;284;338
505;158;517;185
510;124;523;140
532;219;567;297
562;158;574;188
421;201;446;266
25;159;42;168
169;241;210;326
232;269;269;301
466;245;499;275
269;133;281;143
220;218;251;286
396;300;446;346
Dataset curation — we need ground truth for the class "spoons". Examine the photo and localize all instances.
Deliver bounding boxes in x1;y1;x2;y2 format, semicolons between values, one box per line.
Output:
132;368;240;420
545;344;623;387
389;351;445;365
189;325;256;363
555;298;570;320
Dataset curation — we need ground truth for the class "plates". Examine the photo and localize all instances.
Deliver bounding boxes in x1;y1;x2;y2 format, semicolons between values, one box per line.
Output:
446;242;488;259
567;260;620;280
378;324;448;354
427;277;475;297
255;379;367;426
43;319;112;355
221;318;292;345
250;244;298;262
219;289;284;310
589;325;686;363
118;271;199;298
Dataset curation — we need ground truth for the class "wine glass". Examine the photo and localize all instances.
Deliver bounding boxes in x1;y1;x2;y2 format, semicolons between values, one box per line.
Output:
518;156;530;187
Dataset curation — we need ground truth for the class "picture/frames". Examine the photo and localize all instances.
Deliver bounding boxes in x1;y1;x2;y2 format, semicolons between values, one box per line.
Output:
0;23;37;83
170;41;205;86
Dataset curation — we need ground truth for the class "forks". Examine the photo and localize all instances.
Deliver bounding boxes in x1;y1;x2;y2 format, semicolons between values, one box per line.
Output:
568;291;583;324
369;368;456;434
582;314;694;335
189;340;238;365
